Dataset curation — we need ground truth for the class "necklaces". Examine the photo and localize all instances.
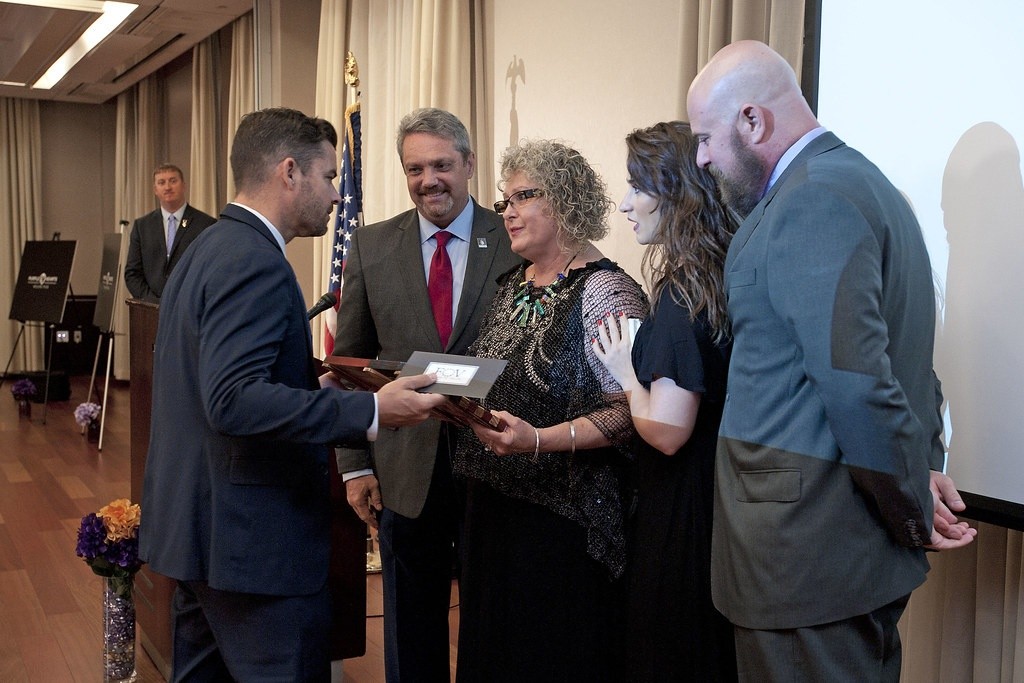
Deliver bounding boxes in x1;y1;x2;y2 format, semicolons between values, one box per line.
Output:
510;247;580;328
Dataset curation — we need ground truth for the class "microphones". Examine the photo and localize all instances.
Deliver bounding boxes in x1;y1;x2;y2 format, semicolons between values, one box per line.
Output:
306;292;338;321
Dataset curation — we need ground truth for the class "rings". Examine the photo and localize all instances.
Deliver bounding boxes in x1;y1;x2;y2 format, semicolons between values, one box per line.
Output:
485;444;491;451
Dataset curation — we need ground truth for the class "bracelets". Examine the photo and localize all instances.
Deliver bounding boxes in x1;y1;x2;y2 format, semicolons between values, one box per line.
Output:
569;421;576;454
528;427;539;462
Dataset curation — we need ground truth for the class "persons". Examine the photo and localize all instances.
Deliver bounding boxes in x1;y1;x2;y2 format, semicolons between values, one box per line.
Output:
688;40;978;683
138;106;446;683
325;107;523;683
449;139;651;683
124;163;218;301
593;120;745;683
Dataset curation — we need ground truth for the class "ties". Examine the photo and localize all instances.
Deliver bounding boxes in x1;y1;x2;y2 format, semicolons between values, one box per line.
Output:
427;231;453;353
166;215;176;261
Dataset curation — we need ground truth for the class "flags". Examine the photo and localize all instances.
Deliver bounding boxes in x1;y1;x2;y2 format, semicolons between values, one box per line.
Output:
326;103;366;365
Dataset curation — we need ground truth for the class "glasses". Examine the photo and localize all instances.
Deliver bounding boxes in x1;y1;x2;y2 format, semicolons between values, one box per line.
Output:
494;190;545;213
